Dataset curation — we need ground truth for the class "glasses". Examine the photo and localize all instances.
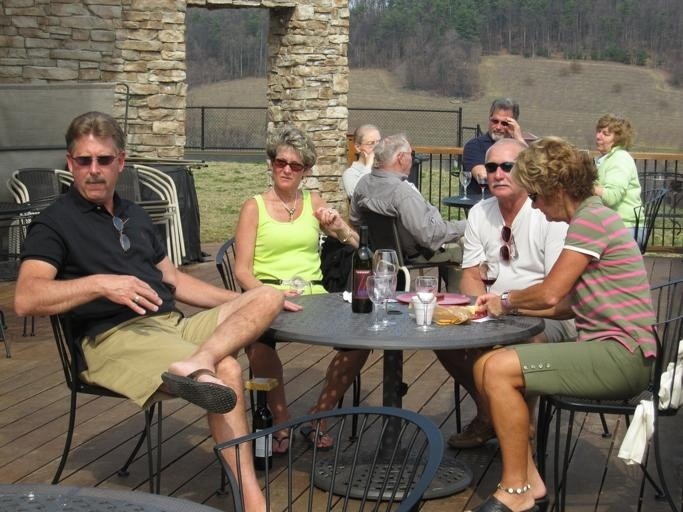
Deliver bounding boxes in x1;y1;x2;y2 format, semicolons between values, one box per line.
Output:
69;155;121;165
360;139;382;146
500;226;512;260
274;157;305;171
490;120;510;126
112;215;132;253
485;162;514;173
528;192;538;202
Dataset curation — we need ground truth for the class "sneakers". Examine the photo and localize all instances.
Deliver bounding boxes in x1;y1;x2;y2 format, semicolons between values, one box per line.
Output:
448;415;497;447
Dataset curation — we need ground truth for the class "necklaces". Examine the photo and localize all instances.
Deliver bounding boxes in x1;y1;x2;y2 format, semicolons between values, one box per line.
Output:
273;188;297;225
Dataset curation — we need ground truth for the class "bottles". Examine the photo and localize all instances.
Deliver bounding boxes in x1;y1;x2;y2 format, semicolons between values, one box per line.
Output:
251;390;273;470
351;225;373;313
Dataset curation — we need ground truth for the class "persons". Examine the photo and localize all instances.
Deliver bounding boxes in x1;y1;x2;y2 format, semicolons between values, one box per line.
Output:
472;134;658;512
593;113;647;250
234;124;370;453
14;112;304;512
349;136;467;293
432;137;569;457
342;124;421;199
459;99;529;219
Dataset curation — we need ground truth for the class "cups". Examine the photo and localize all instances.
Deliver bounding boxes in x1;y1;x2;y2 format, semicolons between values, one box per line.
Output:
409;294;438;325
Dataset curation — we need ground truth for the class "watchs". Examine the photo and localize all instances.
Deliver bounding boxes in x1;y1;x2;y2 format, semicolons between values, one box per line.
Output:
501;289;515;309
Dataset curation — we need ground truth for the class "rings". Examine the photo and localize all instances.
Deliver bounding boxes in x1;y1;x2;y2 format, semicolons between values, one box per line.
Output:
329;210;334;214
135;295;140;302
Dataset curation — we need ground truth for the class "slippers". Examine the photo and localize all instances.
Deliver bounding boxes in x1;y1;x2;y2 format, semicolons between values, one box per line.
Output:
271;435;290;457
161;369;237;414
465;492;549;512
301;426;333;451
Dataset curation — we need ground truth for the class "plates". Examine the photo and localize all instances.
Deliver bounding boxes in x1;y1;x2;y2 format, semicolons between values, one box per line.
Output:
394;291;471;306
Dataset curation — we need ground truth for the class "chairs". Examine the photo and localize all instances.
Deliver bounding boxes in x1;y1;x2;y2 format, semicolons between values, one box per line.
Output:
44;283;229;496
7;162;187;276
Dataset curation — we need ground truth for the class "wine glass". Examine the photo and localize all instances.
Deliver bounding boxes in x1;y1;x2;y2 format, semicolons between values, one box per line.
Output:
459;169;473;200
413;276;438;332
365;248;398;332
477;171;488;202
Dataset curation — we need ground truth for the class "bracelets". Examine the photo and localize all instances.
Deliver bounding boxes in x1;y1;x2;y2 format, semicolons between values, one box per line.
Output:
340;229;352;243
